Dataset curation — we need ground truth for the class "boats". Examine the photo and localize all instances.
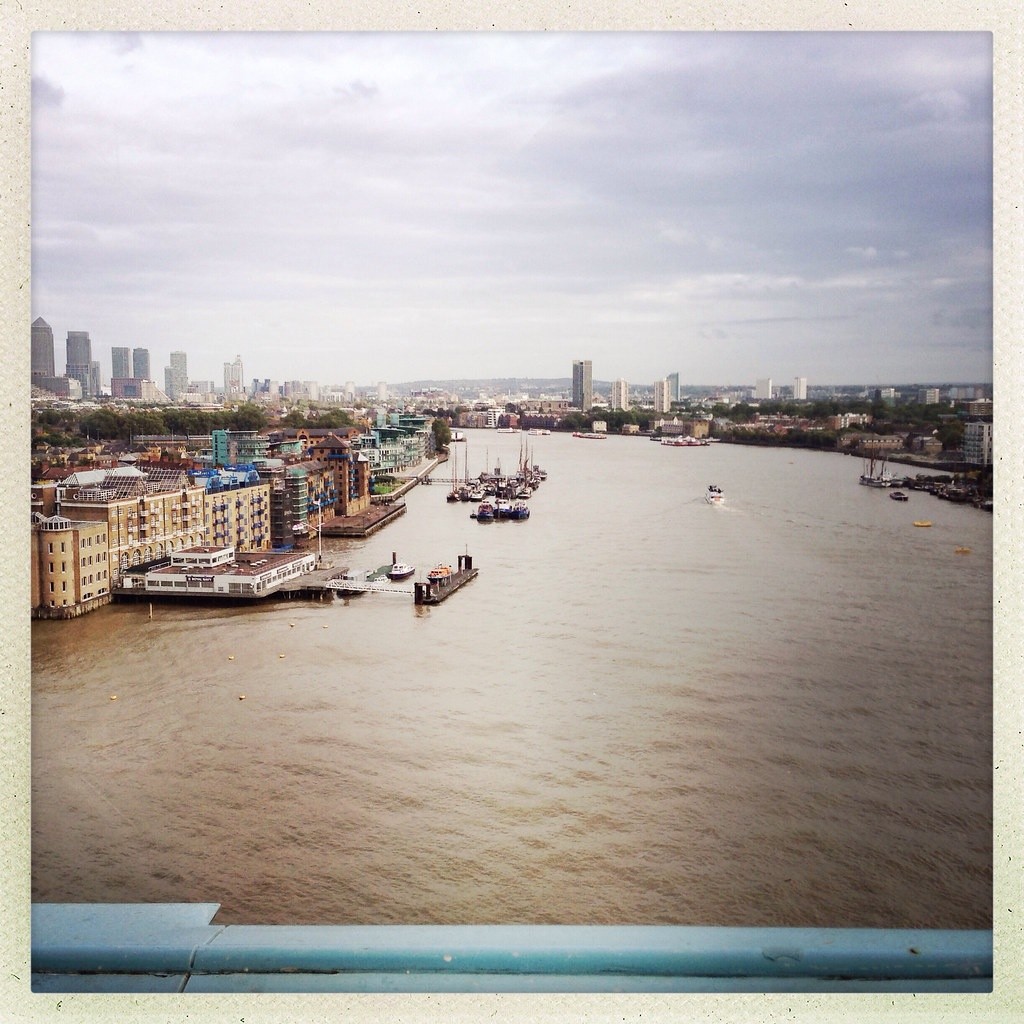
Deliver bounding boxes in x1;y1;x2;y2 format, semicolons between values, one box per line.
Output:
427;563;453;581
446;431;547;522
705;484;725;505
386;563;415;580
660;434;711;447
859;440;994;511
